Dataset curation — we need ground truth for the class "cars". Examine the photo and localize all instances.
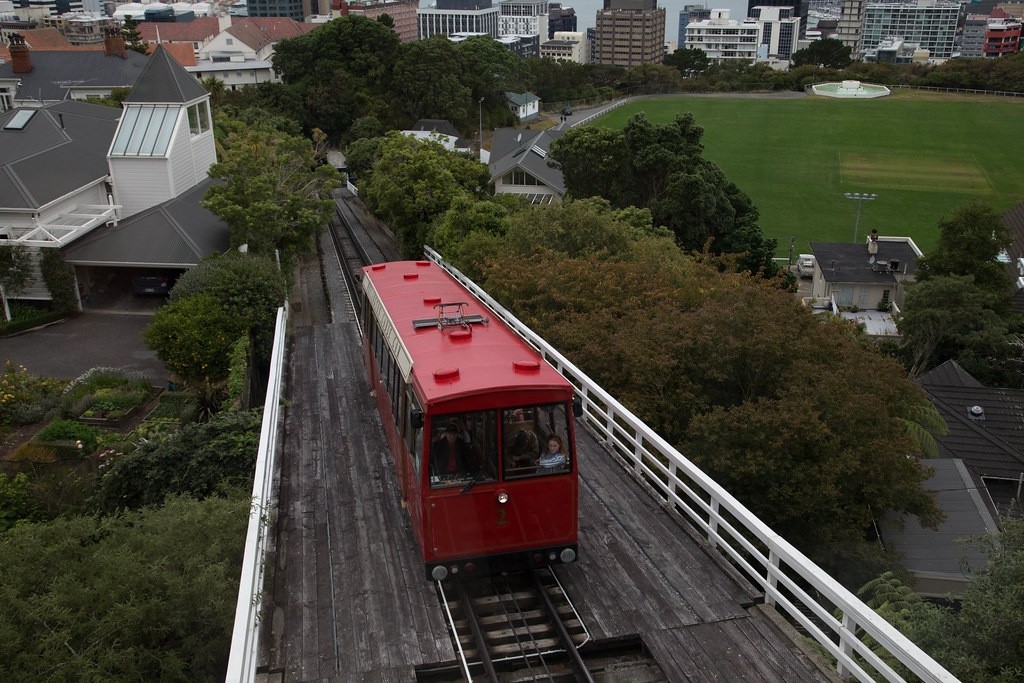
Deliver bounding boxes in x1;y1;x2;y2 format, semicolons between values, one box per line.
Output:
561;108;573;116
134;270;173;301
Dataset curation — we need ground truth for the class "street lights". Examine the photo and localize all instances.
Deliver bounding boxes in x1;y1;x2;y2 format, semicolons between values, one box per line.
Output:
478;95;485;149
843;192;878;244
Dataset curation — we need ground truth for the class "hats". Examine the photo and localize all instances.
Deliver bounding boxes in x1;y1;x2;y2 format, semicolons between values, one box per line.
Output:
516;430;528;444
444;423;459;433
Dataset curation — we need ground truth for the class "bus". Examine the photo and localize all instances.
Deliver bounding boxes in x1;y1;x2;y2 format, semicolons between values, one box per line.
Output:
363;259;585;581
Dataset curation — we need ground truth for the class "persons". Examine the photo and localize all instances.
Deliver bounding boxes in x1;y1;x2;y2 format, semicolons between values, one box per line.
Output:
560;115;566;123
432;424;480;475
511;430;539;467
432;417;470;444
536;434;566;473
